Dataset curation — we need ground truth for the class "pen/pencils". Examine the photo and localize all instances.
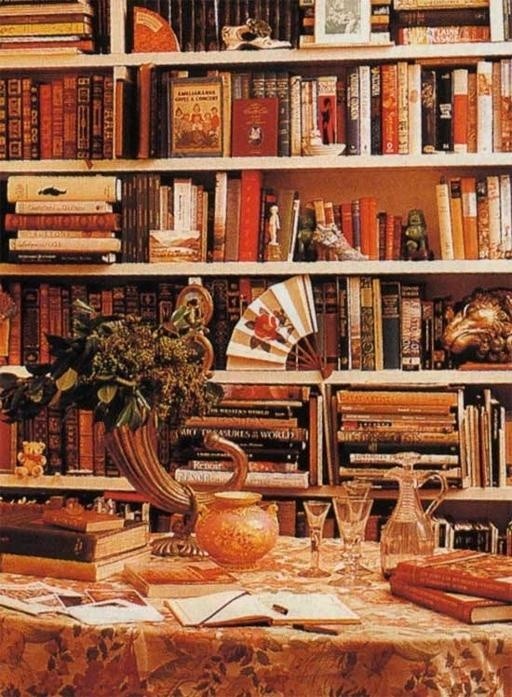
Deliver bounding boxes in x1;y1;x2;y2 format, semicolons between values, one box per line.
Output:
287;623;338;636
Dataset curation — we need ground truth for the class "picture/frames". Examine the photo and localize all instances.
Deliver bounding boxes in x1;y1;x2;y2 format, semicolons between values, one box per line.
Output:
167;77;223;156
314;0;371;45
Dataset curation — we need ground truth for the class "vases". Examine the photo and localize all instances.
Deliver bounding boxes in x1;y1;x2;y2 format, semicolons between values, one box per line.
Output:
196;492;279;573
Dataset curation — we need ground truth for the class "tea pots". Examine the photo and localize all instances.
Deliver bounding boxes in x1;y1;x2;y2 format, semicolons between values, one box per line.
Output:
380;453;449;578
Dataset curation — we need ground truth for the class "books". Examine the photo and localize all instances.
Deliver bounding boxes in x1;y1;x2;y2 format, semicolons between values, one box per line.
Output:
389;575;512;624
163;593;363;629
0;0;512;556
121;560;240;600
0;501;153;582
396;548;512;602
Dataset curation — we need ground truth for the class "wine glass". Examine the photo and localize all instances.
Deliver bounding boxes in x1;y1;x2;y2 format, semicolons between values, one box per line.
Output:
297;498;333;579
329;474;375;592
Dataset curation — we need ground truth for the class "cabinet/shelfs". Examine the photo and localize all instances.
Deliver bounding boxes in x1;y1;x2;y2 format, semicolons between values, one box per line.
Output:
0;0;512;555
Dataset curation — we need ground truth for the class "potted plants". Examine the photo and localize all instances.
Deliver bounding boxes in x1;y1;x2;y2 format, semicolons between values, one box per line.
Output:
0;284;250;553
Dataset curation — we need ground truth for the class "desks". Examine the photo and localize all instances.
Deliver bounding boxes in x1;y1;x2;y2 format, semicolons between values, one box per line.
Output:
0;536;512;697
